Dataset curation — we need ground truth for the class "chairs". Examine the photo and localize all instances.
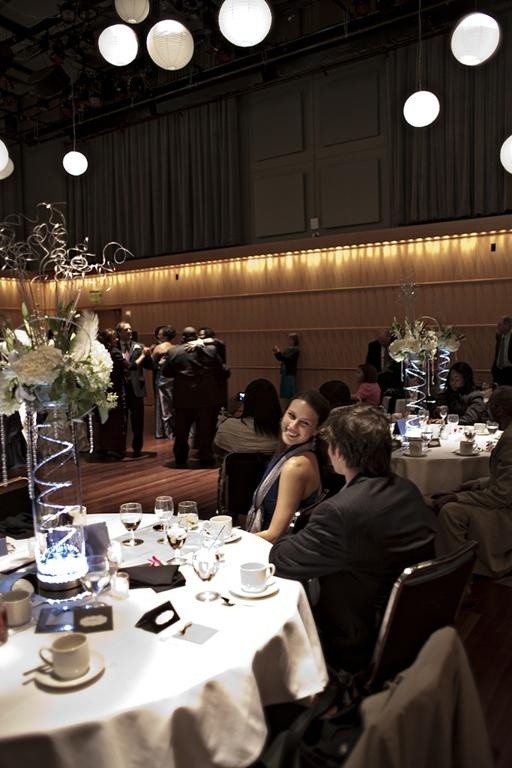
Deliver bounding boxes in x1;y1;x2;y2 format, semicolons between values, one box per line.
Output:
370;538;480;686
0;474;44;539
218;450;273;525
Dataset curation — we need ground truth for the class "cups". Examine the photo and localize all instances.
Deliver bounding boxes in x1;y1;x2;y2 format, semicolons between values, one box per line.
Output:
203;515;233;540
39;634;91;680
1;590;31;626
239;562;275;592
410;441;422;454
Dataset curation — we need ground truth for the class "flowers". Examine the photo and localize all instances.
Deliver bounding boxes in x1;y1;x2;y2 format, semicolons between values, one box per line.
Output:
0;205;135;422
383;303;462;363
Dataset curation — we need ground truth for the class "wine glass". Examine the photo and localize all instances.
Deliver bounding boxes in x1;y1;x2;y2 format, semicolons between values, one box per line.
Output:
417;406;498;452
39;496;220;609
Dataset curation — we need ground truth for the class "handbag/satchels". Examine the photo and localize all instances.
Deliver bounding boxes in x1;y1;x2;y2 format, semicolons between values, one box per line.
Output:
260;668;365;768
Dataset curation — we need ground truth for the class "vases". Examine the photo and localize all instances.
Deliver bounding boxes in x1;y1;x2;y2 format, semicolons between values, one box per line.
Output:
402;353;429;434
27;389;91;589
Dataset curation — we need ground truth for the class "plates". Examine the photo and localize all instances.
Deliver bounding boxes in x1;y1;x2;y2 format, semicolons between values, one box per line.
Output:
34;650;105;689
228;576;279;597
223;528;241;543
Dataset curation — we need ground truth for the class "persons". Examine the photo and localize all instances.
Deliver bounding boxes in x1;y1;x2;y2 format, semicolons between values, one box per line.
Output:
213;379;285;454
366;328;405;387
386;366;437;418
490;315;511;390
246;389;330;543
319;380;351;408
97;321;232;469
435;385;511;579
350;363;382;406
269;401;436;672
426;361;486;425
272;332;300;414
